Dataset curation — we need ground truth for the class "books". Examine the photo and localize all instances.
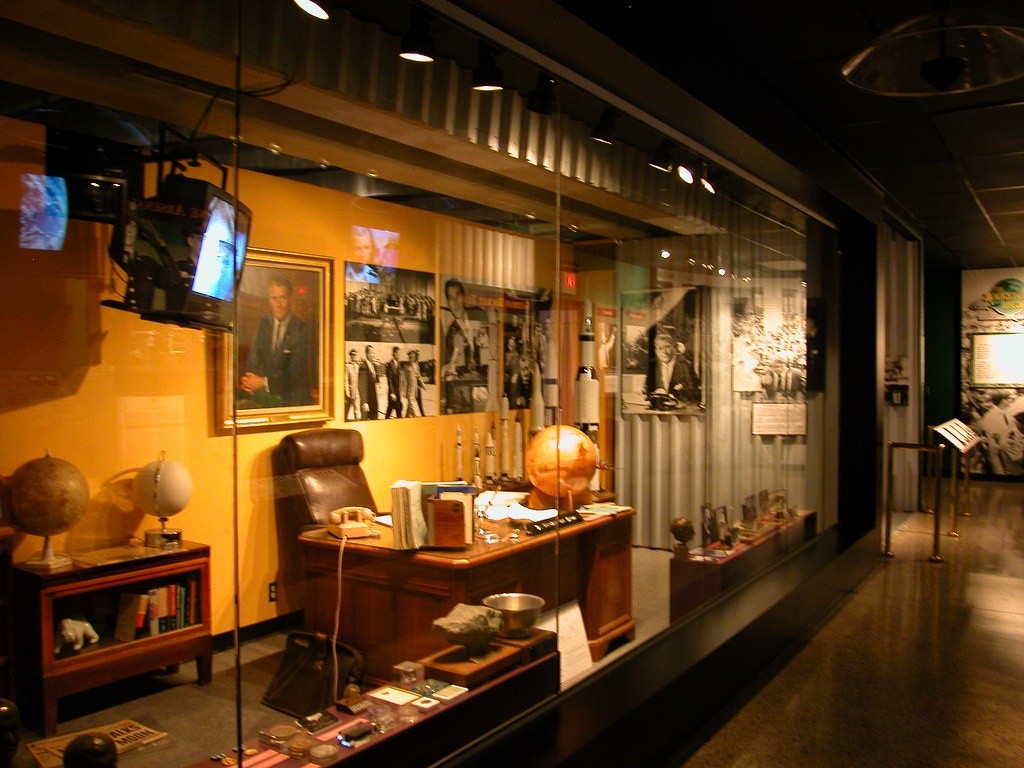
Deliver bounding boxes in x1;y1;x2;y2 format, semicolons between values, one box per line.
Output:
113;574;198;641
391;478;481;551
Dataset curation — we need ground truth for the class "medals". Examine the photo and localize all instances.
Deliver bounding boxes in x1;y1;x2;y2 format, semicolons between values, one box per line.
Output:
244;748;258;756
221;757;237;766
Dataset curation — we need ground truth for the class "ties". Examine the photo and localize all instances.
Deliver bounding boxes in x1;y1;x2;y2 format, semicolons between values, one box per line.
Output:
664;366;670;390
274;323;281;359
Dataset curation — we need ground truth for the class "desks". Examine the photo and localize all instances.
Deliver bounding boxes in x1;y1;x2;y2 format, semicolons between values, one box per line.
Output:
186;652;559;768
670;510;817;622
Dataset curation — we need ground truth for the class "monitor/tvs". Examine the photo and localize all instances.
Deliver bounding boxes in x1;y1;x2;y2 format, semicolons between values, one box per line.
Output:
133;174;253;326
44;125;143;221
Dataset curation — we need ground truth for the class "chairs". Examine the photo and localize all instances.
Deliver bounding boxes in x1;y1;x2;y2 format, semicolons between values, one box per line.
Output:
278;428;390;532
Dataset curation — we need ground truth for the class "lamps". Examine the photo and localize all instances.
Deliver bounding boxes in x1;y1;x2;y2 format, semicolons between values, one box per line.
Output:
526;72;556;115
398;7;436;62
589;106;621;145
295;0;343;21
650;139;728;195
840;0;1024;98
473;39;504;90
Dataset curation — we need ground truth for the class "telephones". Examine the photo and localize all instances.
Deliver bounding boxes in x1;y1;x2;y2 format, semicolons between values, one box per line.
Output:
327;506;381;538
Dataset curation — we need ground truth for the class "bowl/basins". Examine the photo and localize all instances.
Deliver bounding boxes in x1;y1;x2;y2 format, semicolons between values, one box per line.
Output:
481;592;545;638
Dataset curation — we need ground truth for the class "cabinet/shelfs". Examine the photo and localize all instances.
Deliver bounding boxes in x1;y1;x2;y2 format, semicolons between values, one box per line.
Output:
13;539;212;738
298;499;637;696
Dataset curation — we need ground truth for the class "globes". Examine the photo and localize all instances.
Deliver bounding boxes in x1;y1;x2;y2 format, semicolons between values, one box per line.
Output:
5;448;90;570
131;451;193;547
525;424;597;515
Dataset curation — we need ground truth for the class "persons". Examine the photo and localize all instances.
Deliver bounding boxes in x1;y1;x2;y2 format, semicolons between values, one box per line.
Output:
645;332;703;410
443;278;489;407
345;342;430;419
345;283;434;322
506;310;552;404
597;325;616;370
732;306;804;398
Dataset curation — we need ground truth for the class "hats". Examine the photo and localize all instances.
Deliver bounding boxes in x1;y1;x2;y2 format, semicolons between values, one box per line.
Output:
349;348;357;355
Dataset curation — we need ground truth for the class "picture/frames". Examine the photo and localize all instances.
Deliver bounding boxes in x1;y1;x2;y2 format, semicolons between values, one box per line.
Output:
619;286;709;414
214;248;334;431
